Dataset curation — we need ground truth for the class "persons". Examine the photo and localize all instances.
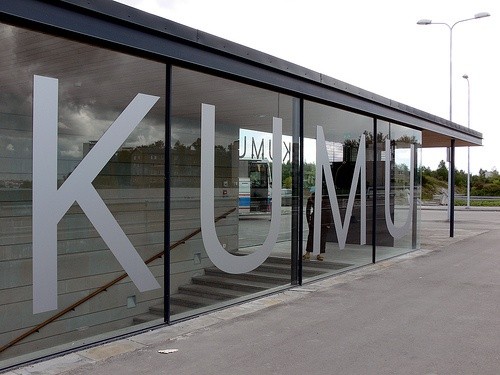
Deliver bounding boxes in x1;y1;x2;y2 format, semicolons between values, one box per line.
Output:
268;182;273;221
302;181;332;262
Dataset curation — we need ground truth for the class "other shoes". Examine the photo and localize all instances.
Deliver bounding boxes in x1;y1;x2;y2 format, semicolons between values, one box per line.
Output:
302;254;310;261
316;254;324;261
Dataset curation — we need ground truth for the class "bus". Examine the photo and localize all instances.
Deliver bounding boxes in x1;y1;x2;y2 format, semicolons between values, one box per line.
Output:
239;156;273;220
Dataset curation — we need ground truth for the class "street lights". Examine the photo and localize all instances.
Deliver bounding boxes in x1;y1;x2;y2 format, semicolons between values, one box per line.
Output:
461;74;470;209
416;11;491;223
